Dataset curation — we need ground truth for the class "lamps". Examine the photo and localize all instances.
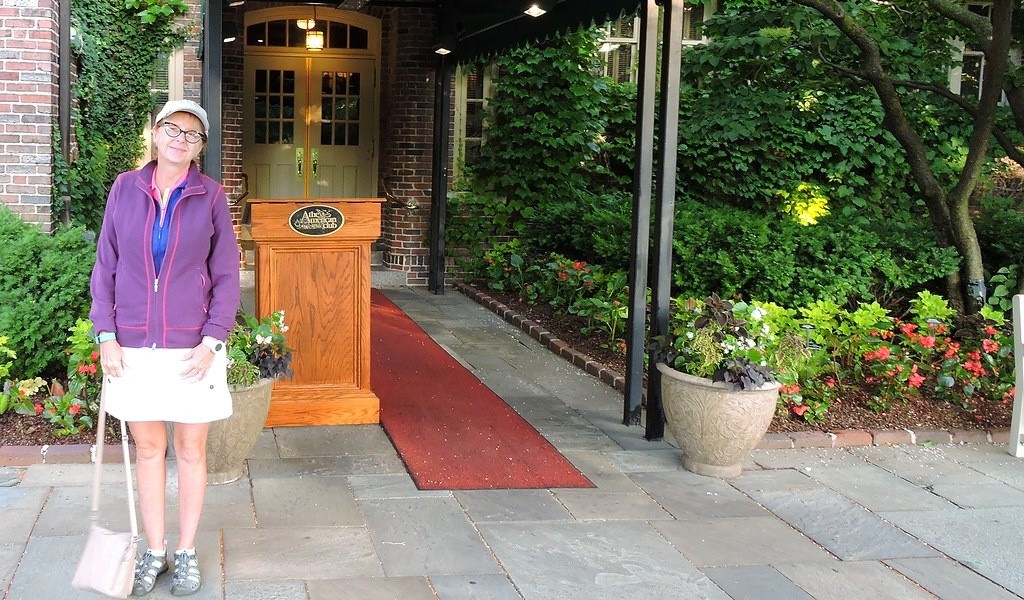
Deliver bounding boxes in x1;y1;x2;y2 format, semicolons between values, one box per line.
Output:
305;6;324;51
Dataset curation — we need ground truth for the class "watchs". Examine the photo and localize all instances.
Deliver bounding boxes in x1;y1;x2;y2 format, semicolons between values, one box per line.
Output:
202;336;225;354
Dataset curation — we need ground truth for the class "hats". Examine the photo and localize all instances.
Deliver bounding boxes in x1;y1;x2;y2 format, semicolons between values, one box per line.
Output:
155;100;210;139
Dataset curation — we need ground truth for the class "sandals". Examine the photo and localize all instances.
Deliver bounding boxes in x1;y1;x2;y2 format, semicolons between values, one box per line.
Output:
132;541;168;596
170;547;200;595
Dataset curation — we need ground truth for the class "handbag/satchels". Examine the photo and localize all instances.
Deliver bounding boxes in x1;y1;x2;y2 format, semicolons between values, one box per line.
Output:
71;525;138;599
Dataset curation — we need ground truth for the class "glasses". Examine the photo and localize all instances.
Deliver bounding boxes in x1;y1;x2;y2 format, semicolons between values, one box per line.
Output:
158;122;206;144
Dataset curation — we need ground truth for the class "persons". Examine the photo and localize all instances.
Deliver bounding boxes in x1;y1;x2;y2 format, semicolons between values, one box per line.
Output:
89;100;241;597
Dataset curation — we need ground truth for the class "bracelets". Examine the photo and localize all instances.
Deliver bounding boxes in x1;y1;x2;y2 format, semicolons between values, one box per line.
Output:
96;333;115;344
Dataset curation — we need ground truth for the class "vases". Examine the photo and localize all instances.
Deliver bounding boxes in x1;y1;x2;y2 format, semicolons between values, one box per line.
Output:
655;362;783;478
167;372;278;486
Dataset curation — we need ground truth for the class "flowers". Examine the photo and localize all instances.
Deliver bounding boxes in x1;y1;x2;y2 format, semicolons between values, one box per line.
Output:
668;294;777;392
227;309;297;385
